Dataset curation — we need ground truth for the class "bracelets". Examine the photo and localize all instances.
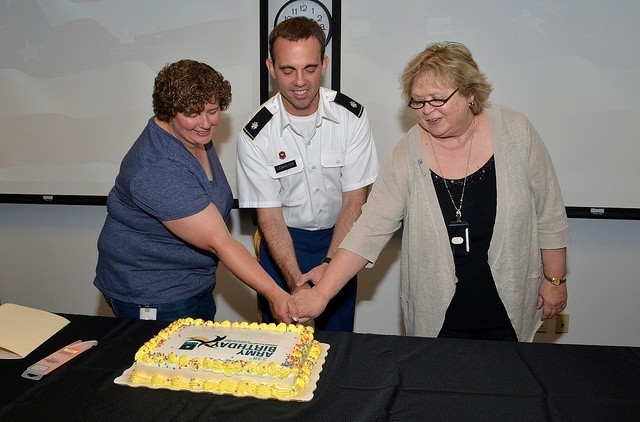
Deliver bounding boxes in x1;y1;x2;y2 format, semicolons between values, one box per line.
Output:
321;258;331;264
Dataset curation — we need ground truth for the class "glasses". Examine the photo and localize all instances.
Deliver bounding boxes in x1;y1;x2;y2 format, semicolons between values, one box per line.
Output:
408;87;459;108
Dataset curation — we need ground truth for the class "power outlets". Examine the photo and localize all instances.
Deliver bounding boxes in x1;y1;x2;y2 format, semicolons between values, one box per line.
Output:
555;313;569;333
537;319;548;333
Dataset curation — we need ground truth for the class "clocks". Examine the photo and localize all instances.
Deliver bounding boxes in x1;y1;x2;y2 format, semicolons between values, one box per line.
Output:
273;0;332;47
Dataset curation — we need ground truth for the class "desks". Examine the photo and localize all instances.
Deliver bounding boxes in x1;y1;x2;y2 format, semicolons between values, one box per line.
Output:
0;310;640;421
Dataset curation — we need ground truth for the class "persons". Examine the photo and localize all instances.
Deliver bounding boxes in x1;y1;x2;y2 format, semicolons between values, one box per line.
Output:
290;40;568;343
236;16;380;332
92;59;299;323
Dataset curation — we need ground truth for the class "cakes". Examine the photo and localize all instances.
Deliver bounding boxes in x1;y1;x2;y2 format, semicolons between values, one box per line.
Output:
131;318;320;398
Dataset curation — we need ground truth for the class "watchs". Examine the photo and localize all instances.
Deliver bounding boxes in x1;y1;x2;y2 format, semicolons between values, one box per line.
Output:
543;273;567;286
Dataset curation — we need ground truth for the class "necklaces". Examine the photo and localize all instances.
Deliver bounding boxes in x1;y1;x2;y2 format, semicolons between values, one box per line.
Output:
427;116;476;219
